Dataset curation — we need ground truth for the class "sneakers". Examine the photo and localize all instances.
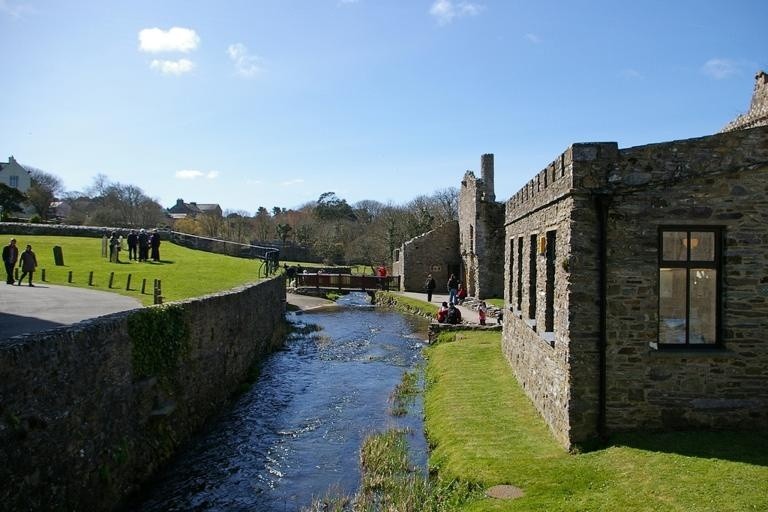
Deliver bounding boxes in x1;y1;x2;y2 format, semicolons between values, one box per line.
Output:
29;283;35;287
109;259;121;263
6;280;16;285
128;259;160;265
18;280;21;286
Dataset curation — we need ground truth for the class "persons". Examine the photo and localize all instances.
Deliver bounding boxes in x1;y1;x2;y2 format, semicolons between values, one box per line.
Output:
294;264;304;286
476;300;483;312
436;302;448;322
481;302;486;313
286;264;296;287
438;301;462;323
107;227;161;264
455;283;466;305
1;238;18;286
424;273;437;302
377;262;388;291
479;309;486;326
496;308;504;325
447;274;459;304
17;244;38;287
